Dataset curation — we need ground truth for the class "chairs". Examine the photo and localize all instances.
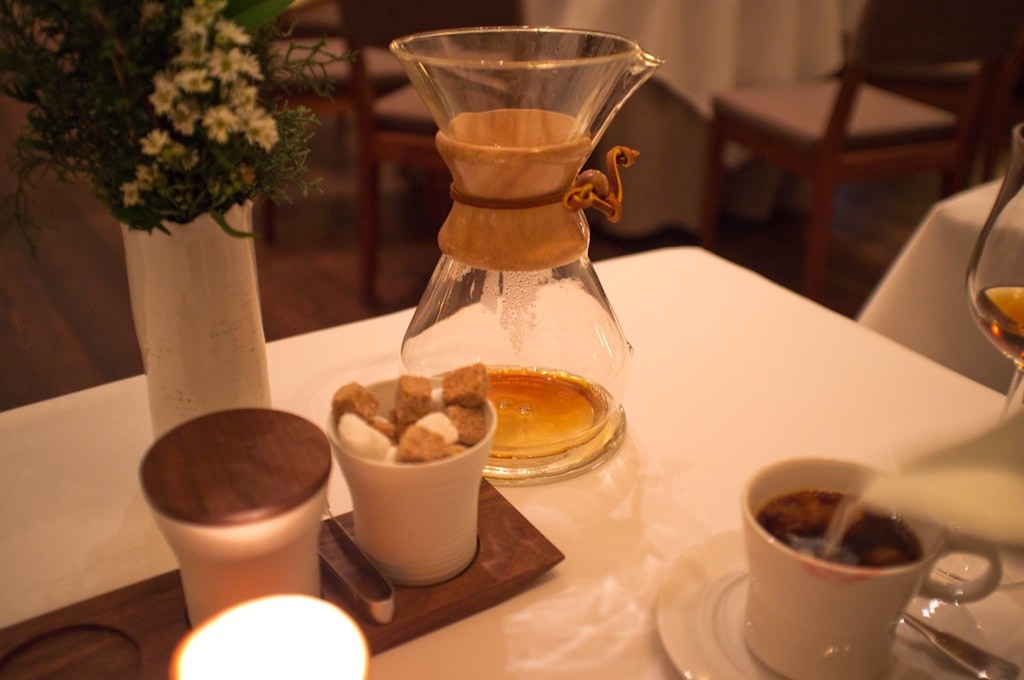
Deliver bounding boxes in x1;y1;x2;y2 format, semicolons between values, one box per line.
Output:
336;0;535;313
706;2;1023;308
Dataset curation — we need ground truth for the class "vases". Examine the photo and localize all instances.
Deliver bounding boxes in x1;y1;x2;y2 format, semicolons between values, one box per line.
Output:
116;201;273;445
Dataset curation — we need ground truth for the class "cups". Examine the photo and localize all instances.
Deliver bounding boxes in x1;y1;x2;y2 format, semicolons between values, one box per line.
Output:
329;377;497;587
743;459;1001;680
139;406;331;630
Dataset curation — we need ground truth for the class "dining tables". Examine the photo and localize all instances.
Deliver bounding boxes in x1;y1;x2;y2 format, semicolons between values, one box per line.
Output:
1;240;1024;680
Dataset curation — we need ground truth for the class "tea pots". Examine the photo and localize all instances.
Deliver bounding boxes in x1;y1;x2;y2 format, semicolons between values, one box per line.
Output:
390;28;662;483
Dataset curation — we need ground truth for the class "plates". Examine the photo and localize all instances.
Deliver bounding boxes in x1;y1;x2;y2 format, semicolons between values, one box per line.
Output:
655;520;1024;680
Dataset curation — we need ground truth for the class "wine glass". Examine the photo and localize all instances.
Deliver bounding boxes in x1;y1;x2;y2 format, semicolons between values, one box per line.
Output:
963;124;1024;428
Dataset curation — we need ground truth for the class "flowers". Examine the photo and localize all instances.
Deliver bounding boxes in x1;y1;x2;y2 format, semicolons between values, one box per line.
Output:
1;0;363;244
